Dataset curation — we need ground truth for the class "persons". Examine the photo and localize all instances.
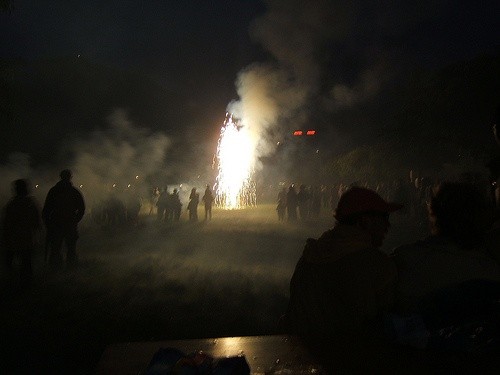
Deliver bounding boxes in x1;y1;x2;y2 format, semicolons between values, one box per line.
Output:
0;180;41;265
394;180;500;330
278;185;408;334
275;172;500;226
41;170;85;260
85;181;213;228
